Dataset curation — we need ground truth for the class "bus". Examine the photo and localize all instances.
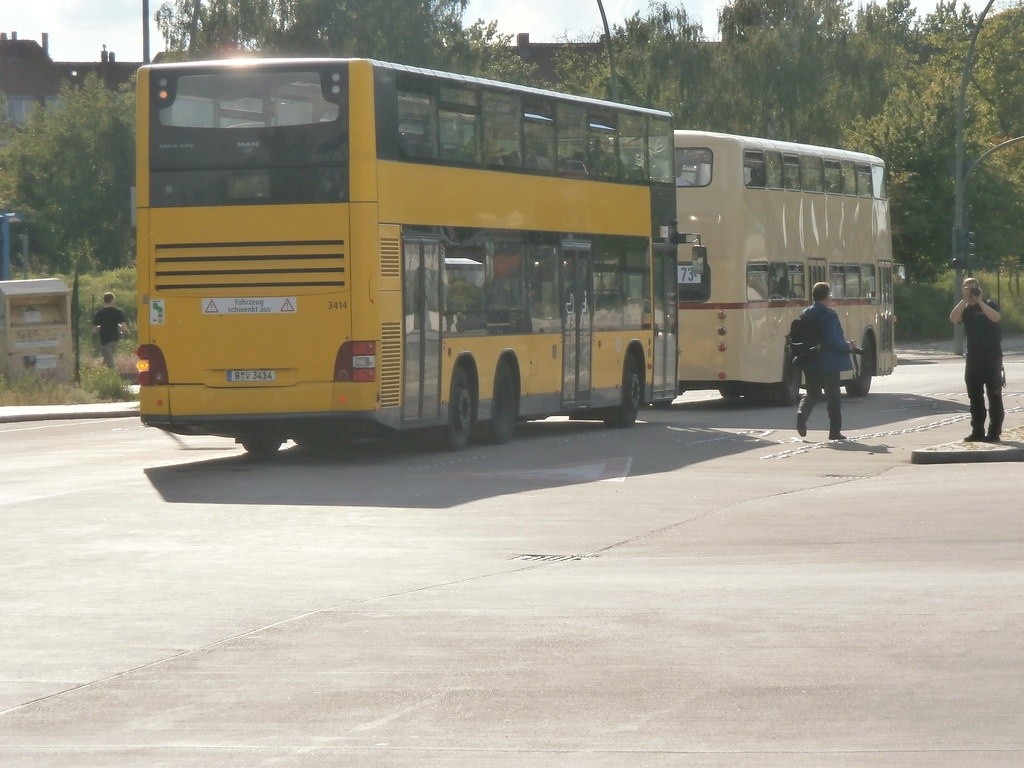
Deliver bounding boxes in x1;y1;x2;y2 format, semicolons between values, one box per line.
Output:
133;54;709;457
604;129;904;408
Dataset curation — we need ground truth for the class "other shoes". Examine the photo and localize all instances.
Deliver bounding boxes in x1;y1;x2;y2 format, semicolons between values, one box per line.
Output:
829;431;847;440
965;431;986;442
982;432;1000;442
796;411;807;438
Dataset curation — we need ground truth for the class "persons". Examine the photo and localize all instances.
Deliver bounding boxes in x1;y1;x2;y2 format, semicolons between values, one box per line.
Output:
747;170;758;185
93;293;128;367
591;138;605;155
796;282;856;440
950;278;1003;442
534;144;553;169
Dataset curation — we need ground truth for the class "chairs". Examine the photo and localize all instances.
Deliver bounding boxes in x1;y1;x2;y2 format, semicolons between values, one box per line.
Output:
396;130;645;182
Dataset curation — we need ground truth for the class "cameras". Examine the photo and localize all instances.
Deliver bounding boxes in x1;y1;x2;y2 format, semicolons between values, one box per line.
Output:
970;286;981;297
849;347;865;356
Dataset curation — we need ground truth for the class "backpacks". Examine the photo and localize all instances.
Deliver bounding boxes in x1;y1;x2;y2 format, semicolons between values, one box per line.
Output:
790;307;822;367
